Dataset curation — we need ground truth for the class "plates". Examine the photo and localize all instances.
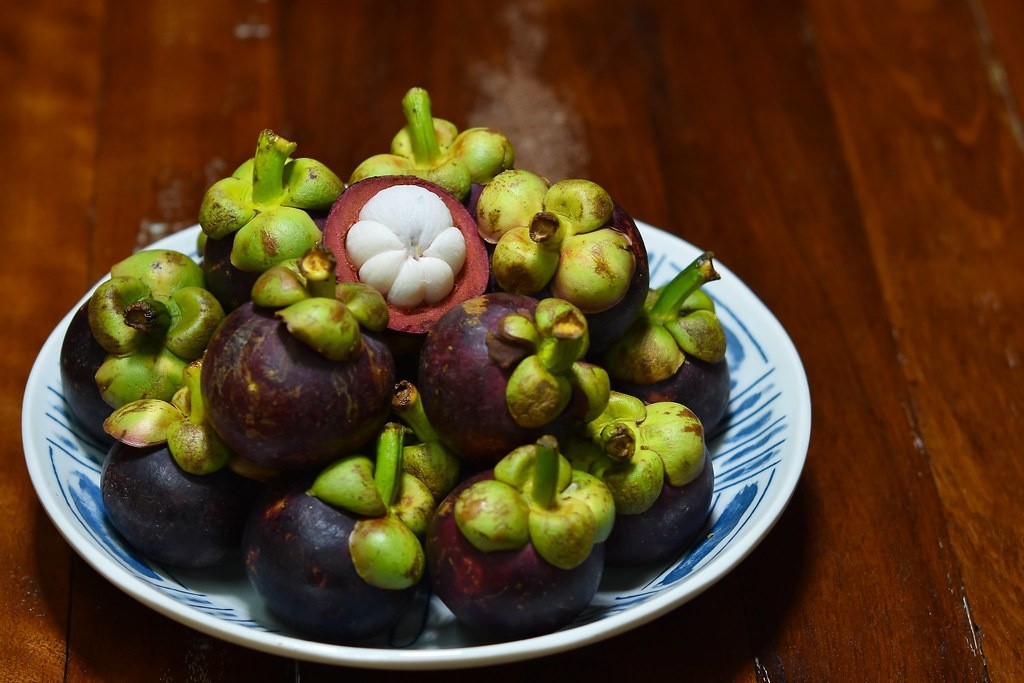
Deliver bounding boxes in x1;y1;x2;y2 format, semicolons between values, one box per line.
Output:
21;221;812;670
